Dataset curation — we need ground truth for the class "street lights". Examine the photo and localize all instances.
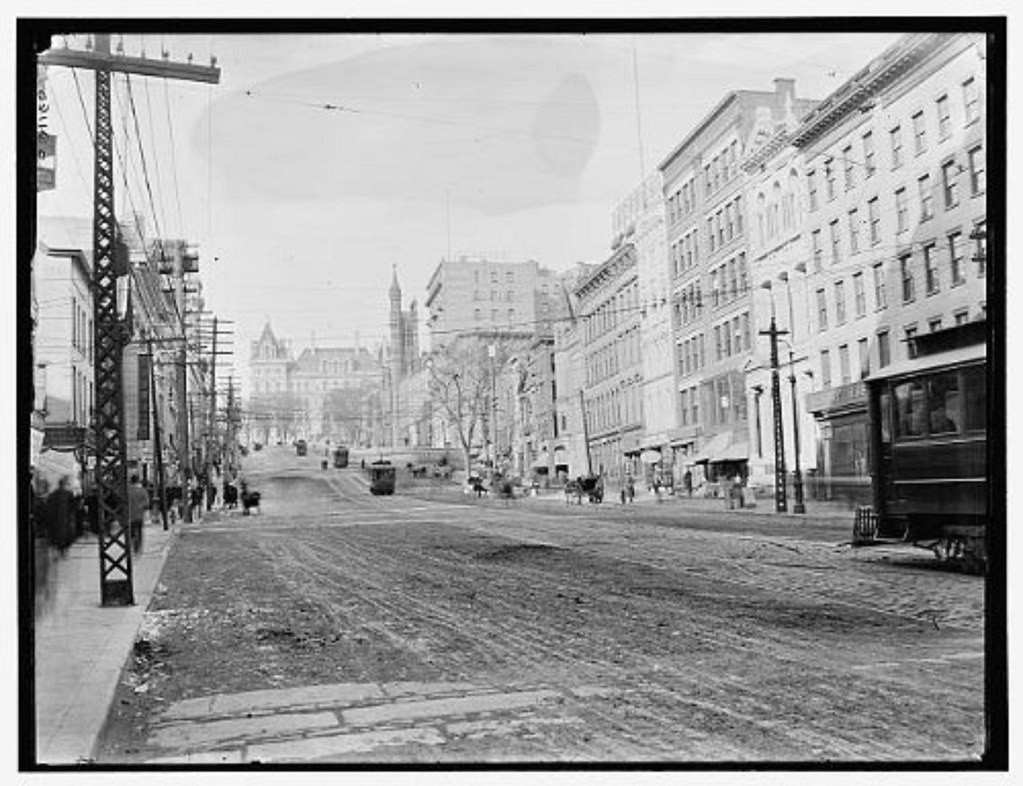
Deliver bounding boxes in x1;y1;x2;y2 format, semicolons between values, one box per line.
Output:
777;336;809;513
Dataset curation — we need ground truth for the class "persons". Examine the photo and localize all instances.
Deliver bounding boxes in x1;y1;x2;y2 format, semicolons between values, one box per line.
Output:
629;484;635;502
147;484;154;498
735;472;741;485
34;474;78;550
86;487;99;534
129;475;150;553
211;483;216;502
621;489;626;503
165;486;182;507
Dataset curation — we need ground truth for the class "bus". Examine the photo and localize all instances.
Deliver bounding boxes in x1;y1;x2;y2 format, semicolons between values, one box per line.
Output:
865;315;985;565
295;438;308;456
333;444;349;469
370;460;397;494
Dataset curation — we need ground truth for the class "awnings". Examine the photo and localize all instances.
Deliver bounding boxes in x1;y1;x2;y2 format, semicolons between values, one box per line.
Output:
695;433;747;465
